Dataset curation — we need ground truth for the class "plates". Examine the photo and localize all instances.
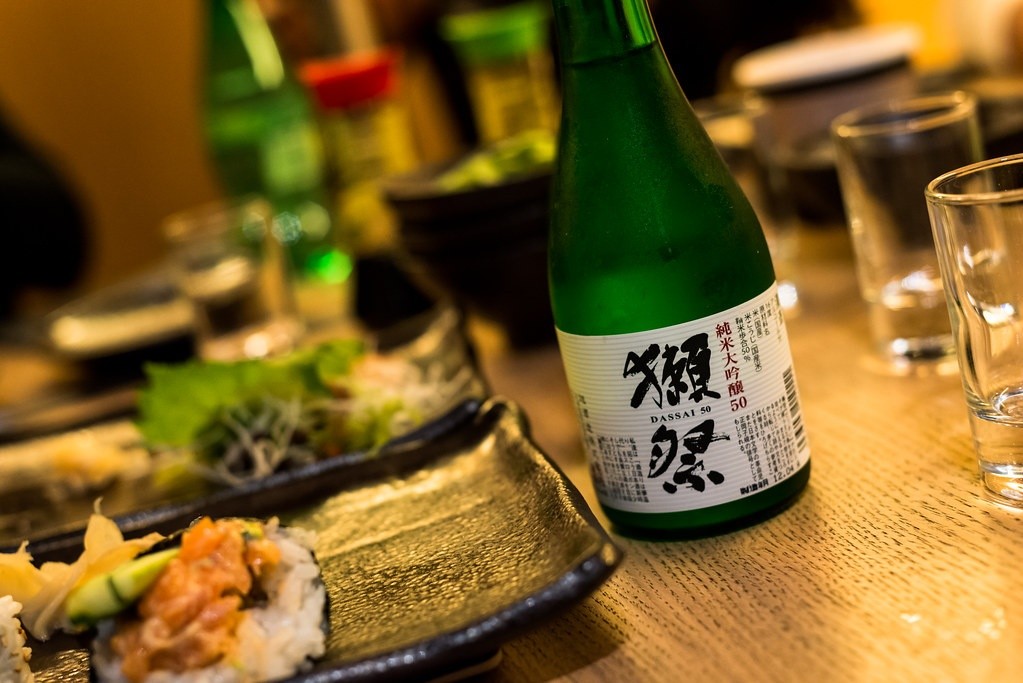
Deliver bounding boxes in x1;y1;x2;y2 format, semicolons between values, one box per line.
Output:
1;395;627;683
780;110;1023;200
0;308;492;552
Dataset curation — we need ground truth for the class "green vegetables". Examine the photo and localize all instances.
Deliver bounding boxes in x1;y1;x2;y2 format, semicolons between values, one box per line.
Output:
130;338;424;483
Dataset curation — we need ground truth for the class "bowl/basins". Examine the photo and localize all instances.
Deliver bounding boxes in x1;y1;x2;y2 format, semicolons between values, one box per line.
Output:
388;96;766;351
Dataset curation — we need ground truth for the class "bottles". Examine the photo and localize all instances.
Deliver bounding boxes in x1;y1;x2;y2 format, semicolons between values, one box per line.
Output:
299;52;452;345
193;0;346;283
544;0;815;540
445;6;554;141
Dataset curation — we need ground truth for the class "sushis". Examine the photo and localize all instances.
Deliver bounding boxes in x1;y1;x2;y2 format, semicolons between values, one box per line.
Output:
83;515;332;683
0;593;36;683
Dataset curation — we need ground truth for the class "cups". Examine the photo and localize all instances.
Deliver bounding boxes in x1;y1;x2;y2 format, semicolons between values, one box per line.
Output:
924;151;1023;512
831;89;1022;382
157;196;294;368
